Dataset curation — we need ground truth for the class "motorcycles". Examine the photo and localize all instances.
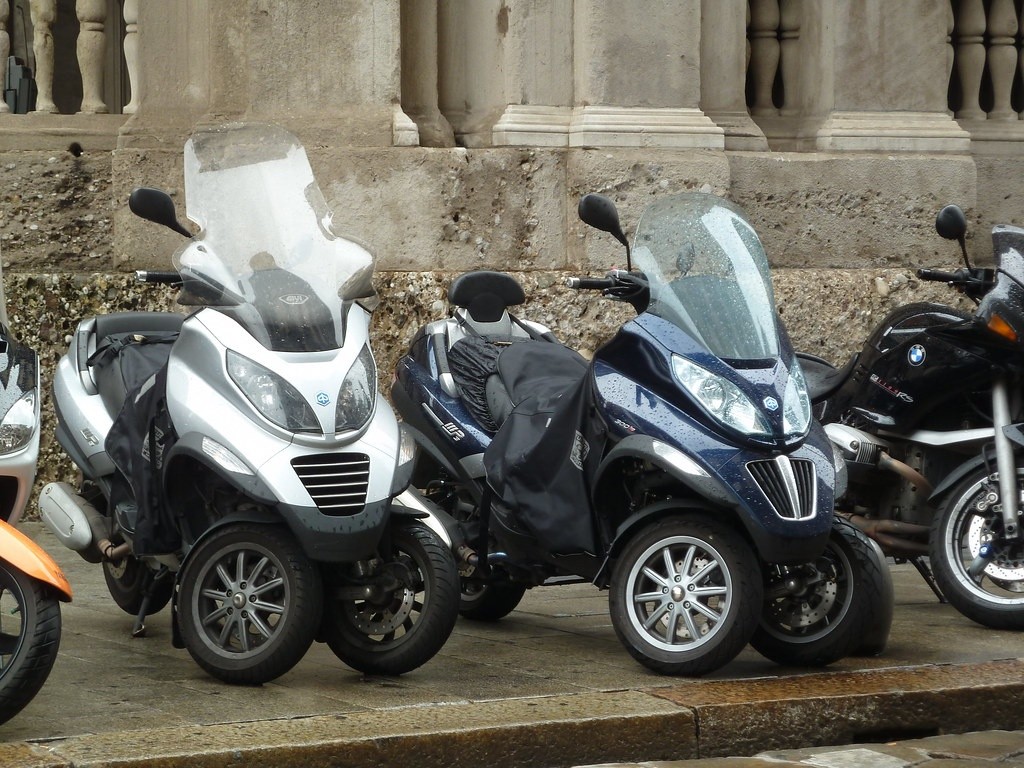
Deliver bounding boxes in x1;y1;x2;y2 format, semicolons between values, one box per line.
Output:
36;127;459;687
392;192;894;678
0;293;73;728
790;207;1024;630
